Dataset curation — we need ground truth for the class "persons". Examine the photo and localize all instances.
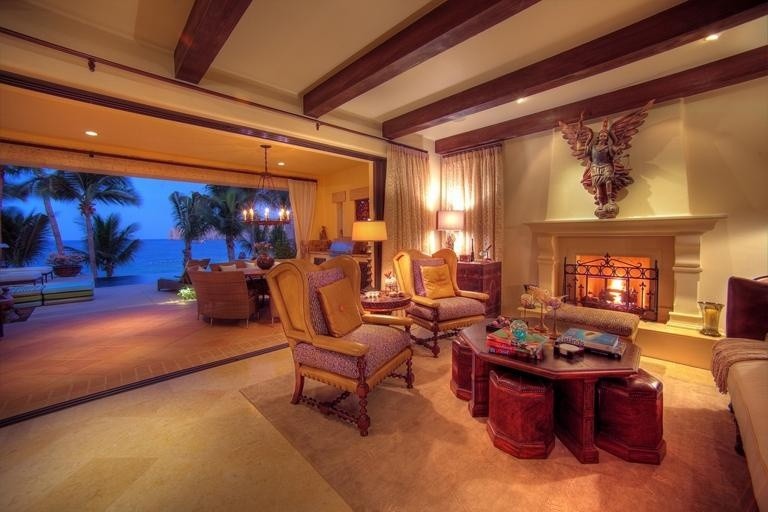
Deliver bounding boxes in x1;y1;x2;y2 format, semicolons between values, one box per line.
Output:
587;129;625;210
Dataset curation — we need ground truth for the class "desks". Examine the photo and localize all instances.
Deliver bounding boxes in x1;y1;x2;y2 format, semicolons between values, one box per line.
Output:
359;290;412;331
517;301;553;325
452;318;643;464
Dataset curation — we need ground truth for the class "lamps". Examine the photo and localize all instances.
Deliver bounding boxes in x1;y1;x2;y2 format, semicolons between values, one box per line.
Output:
237;144;290;226
352;218;389;289
436;204;466;249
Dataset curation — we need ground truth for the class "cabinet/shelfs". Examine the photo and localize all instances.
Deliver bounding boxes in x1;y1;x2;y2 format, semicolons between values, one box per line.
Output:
458;256;501;318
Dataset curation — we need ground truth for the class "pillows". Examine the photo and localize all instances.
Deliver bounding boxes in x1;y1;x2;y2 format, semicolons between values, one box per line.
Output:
198;265;204;272
418;262;457;299
314;276;364;339
244;262;257;268
219;264;236;271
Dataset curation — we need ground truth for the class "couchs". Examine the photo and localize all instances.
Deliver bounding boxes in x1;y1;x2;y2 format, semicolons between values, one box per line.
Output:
711;274;768;511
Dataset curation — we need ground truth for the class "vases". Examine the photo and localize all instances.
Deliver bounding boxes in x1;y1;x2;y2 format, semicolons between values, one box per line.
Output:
698;300;725;337
520;306;529;325
54;263;82;277
256;252;275;270
549;308;562;340
534;303;548;331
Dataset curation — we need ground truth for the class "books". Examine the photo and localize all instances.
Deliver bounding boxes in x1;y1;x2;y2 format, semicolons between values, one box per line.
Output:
486;327;628;360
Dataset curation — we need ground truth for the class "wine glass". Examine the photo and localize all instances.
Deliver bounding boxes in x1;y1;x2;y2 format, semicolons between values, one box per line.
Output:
534;290;550;331
546;296;563;339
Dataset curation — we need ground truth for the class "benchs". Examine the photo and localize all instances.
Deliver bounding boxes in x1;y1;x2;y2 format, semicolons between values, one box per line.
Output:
547;302;640;347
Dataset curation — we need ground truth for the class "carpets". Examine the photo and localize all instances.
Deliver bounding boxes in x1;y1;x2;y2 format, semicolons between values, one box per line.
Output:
238;364;755;510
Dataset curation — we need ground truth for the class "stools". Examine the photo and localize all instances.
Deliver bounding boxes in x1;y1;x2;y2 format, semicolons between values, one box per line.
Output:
451;334;474;402
594;366;668;465
487;367;555;460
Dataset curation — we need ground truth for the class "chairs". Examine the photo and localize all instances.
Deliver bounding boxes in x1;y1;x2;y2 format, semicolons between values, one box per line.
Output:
187;269;212;326
393;248;490;358
212;270;259;328
266;255;416;437
2;267;54;282
175;257;210;287
0;270;43;287
207;261;245;270
157;259;199;291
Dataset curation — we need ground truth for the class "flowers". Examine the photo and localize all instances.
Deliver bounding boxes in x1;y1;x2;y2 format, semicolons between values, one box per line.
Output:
534;287;550;303
45;250;87;265
253;240;273;253
544;295;562;310
521;292;534;309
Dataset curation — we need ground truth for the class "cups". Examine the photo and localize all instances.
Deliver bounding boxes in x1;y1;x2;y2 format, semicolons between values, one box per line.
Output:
524;285;537;309
366;292;377;300
385;280;397;296
